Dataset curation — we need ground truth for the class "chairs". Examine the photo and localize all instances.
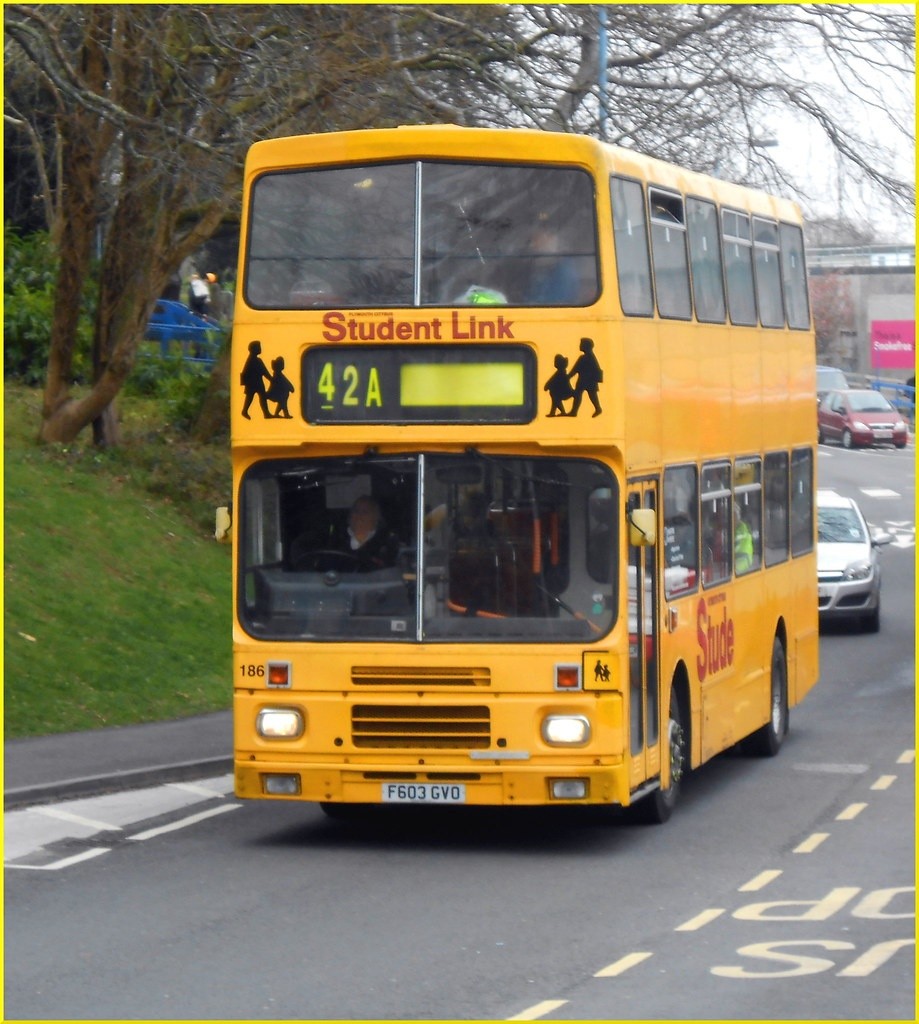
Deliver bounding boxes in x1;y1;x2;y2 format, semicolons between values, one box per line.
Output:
485;499;556;551
306;506;385;546
288;280;342;306
454;538;500;614
488;539;516;619
504;541;534;617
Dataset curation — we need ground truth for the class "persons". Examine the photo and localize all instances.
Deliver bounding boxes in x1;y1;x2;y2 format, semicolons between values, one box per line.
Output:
734;501;753;574
528;220;581;304
188;272;224;361
313;494;391;576
451;492;495;546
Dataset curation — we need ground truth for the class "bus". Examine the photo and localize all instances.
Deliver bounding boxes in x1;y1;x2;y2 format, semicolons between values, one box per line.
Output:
184;124;823;831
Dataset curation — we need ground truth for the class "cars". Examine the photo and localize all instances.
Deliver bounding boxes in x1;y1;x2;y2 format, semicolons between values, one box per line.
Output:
817;388;908;449
817;495;893;634
